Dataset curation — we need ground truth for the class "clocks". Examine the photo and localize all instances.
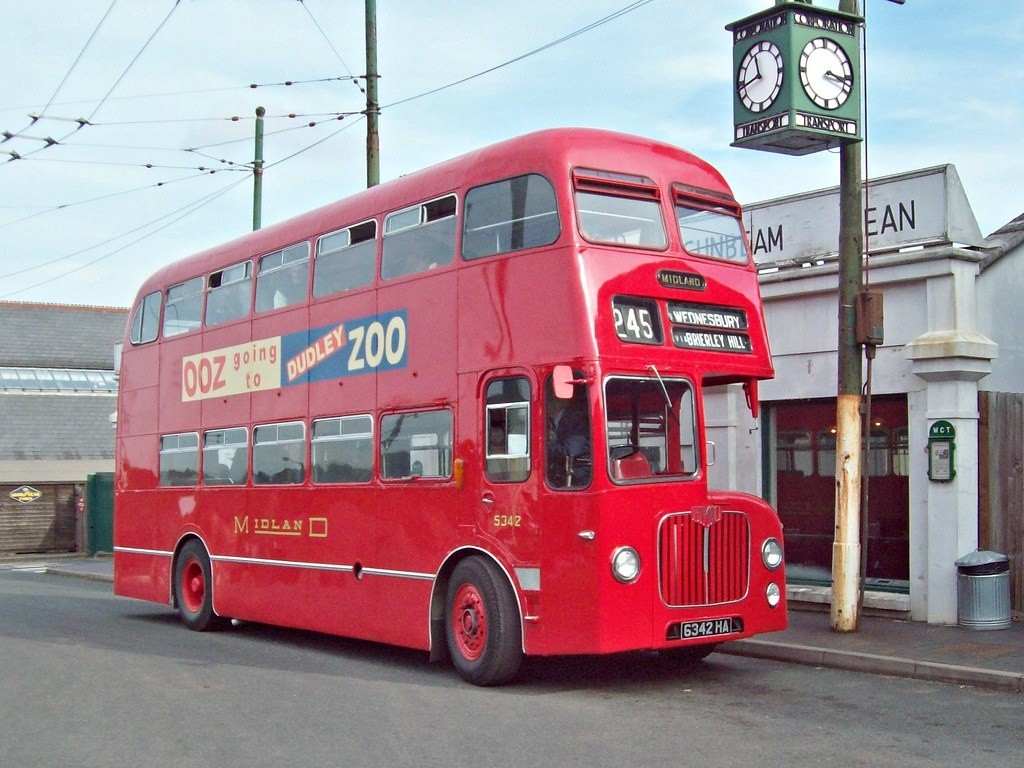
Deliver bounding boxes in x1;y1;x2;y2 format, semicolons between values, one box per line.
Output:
798;36;854;111
736;40;784;114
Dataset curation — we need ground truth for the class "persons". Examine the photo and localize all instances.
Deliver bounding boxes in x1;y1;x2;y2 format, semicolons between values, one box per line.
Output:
542;391;587;492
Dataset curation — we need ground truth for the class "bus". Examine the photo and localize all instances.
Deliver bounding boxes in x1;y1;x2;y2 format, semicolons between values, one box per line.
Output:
111;127;790;686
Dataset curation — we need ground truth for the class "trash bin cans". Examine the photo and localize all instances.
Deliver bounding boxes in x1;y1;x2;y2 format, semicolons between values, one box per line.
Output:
954;547;1013;632
